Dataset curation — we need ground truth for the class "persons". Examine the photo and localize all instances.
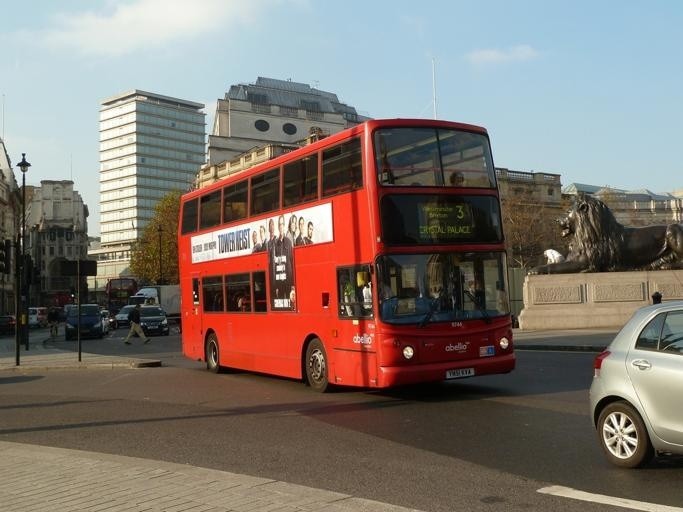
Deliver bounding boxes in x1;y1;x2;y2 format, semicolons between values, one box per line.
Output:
296;216;305;246
124;304;151;345
274;216;295;299
450;171;464;186
260;226;267;252
363;264;393;313
47;308;60;337
252;231;261;253
286;214;297;246
267;219;276;252
303;221;313;245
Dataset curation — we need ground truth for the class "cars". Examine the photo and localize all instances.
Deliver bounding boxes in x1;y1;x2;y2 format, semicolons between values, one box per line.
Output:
0;278;170;341
589;301;682;468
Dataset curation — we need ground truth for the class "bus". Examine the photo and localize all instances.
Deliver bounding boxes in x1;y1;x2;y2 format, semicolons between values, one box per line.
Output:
176;119;516;392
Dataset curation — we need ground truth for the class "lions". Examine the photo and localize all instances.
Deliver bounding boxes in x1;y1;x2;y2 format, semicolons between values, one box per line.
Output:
526;192;682;277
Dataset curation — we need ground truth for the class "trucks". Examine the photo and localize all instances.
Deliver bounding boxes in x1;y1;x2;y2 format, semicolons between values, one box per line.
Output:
128;284;181;321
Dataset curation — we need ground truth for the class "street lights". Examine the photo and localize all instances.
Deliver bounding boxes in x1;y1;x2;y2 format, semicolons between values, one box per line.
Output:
157;226;163;285
15;154;30;366
